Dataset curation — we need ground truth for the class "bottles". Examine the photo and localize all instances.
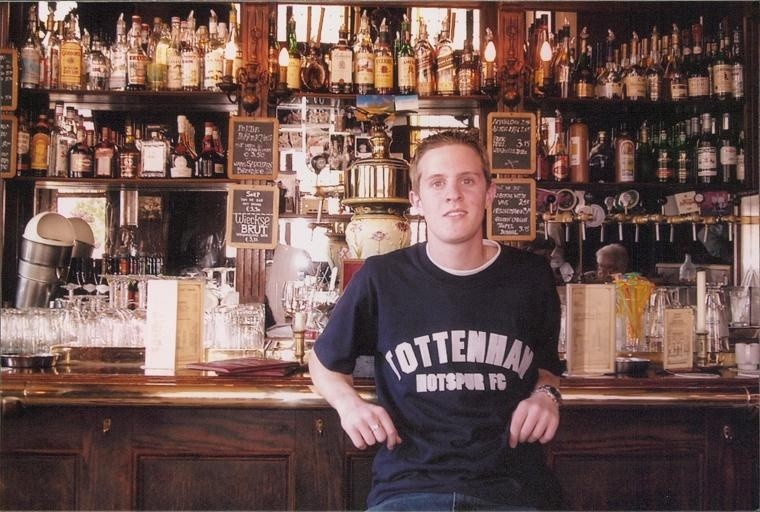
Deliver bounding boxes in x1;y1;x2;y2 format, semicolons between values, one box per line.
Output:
14;100;228;180
267;4;498;99
50;188;236;294
527;105;750;186
527;9;747;103
649;285;731;375
18;5;237;92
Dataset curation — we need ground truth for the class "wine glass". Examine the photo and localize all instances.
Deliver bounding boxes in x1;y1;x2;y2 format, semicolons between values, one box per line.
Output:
56;266;240;356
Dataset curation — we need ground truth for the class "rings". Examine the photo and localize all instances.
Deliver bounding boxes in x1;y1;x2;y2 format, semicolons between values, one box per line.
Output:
372;423;381;433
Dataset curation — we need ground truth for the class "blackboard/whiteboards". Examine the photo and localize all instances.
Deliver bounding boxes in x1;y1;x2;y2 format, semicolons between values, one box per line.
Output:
227;185;278;249
228;117;279;180
487;179;536;237
487;112;536;174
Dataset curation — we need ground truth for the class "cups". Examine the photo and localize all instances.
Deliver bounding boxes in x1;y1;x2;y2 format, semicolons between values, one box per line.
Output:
2;306;56;356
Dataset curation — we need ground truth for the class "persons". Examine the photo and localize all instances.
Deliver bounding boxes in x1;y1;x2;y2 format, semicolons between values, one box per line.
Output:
524;233;556;263
595;241;630;283
307;129;567;512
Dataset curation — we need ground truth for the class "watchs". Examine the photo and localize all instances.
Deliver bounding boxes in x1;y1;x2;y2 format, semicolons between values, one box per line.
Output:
533;383;565;406
